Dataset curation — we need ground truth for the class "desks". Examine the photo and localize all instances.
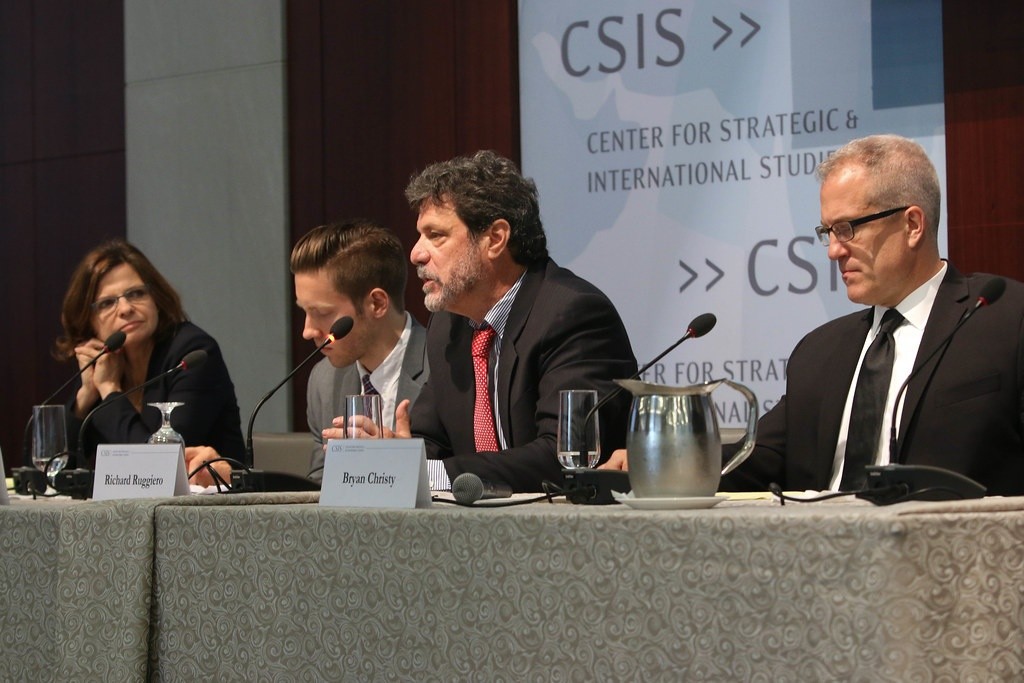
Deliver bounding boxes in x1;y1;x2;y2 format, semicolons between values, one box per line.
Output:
0;481;1024;683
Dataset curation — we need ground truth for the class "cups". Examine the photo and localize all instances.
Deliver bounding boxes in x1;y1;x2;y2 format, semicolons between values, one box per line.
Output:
343;395;383;440
557;390;601;469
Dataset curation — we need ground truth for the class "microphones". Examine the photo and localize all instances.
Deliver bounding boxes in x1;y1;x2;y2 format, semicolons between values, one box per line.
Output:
865;277;1009;502
55;350;208;498
561;314;718;505
452;474;513;505
230;316;354;493
11;331;126;496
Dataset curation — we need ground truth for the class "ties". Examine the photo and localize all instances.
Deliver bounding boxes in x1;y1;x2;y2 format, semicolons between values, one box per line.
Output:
471;328;500;453
359;375;384;429
838;308;904;493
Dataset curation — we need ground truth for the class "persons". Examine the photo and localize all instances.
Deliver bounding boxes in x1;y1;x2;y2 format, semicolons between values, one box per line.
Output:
321;146;640;497
181;220;431;487
21;240;246;470
595;133;1024;494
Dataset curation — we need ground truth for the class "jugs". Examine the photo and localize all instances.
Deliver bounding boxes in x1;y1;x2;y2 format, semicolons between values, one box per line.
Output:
614;378;759;498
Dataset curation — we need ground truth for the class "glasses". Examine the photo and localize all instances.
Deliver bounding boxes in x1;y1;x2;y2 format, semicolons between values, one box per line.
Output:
815;206;910;246
93;285;150;311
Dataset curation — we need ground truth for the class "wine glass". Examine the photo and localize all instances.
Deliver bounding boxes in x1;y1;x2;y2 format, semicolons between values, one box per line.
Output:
146;402;186;459
31;405;68;495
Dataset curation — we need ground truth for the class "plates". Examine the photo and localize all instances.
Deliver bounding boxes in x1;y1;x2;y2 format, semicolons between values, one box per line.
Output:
615;497;729;508
